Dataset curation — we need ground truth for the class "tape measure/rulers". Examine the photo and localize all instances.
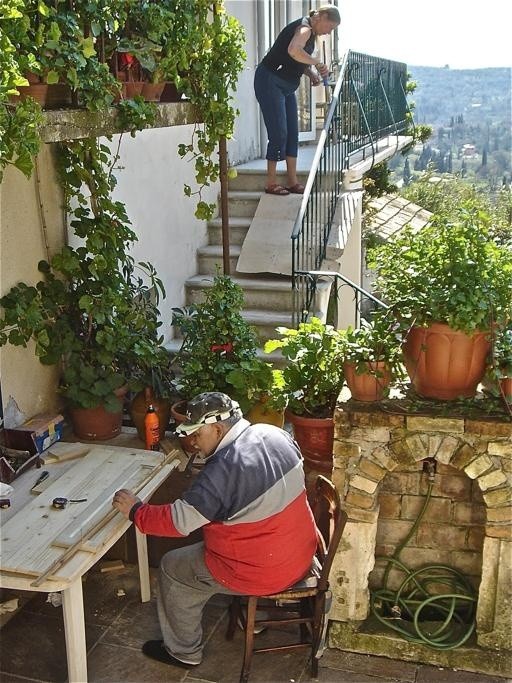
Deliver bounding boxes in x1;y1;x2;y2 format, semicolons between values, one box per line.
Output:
53;498;68;509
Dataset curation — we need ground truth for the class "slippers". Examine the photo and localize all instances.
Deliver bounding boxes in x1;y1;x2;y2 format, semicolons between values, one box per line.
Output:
265;184;289;194
285;184;304;194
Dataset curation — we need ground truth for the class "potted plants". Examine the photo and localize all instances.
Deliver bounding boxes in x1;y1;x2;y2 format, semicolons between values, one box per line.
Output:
3;196;147;441
393;174;502;402
169;269;254;457
340;322;398;405
122;264;168;446
264;321;351;460
1;3;225;115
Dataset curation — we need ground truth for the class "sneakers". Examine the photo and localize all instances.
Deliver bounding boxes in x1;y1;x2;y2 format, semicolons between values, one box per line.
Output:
238;616;265;633
142;640;198;668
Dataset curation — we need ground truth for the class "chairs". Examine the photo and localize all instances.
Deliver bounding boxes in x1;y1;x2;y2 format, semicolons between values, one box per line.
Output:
225;473;348;683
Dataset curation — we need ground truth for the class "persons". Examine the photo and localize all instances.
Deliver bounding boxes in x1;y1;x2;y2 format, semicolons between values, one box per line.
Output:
253;4;341;195
111;388;318;669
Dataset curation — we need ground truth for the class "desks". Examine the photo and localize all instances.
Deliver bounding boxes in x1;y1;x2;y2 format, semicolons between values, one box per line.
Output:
0;440;181;683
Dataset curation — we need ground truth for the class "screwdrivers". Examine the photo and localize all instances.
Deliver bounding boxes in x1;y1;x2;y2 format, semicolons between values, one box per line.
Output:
31;471;48;491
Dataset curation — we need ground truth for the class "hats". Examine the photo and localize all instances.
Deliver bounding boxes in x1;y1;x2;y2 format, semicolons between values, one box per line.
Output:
172;392;235;438
